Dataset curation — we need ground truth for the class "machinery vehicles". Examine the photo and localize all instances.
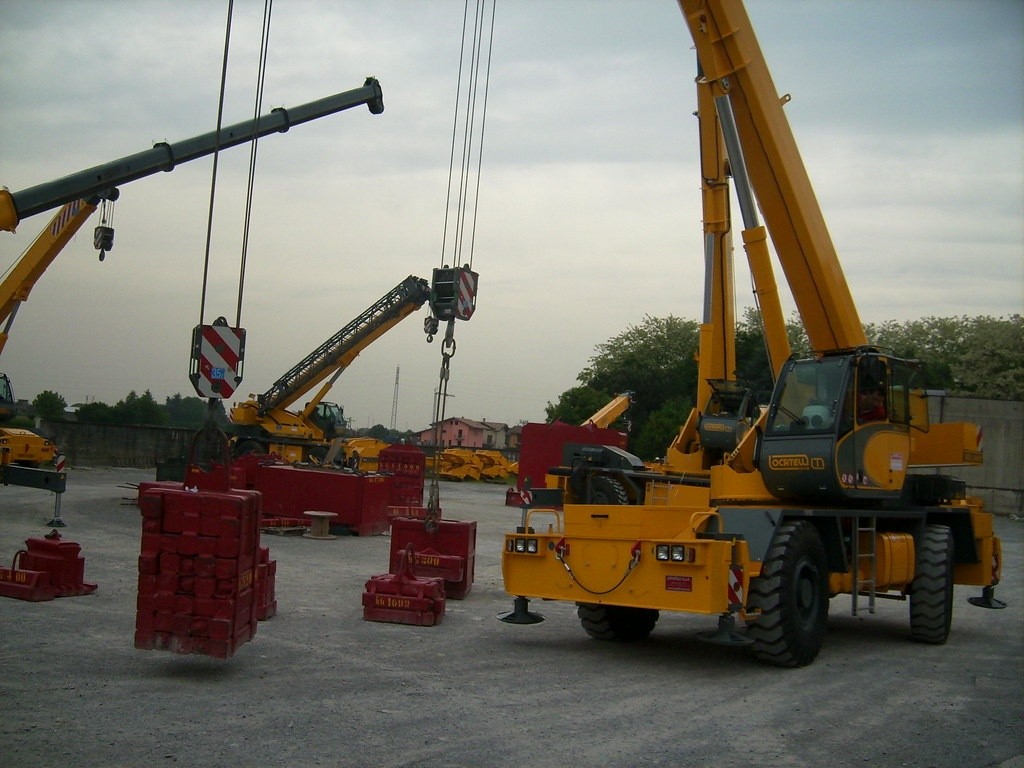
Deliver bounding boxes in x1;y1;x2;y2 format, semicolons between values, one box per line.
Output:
153;273;438;531
0;73;384;528
185;0;1012;670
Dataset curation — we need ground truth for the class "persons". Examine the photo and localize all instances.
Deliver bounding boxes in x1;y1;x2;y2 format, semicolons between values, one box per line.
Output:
851;380;886;431
